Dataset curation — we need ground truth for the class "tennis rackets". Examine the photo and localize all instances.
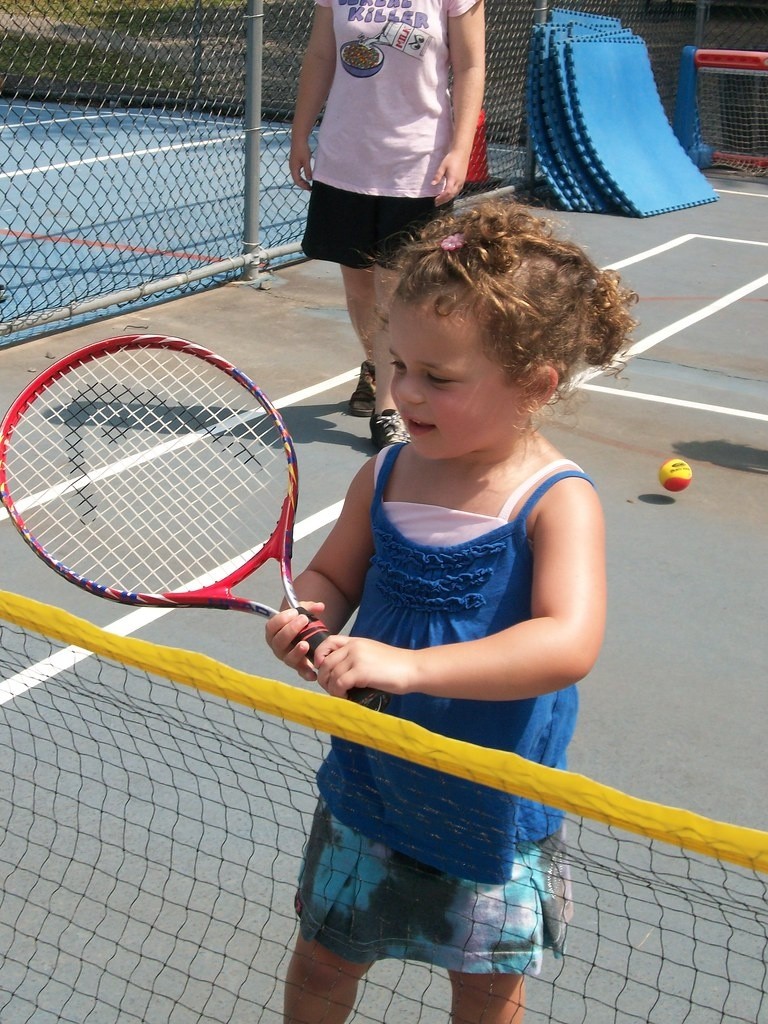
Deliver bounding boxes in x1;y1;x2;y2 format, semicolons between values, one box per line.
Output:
0;332;394;715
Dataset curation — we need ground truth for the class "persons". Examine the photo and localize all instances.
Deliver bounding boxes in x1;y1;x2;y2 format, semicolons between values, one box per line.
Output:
264;201;639;1024
287;0;485;452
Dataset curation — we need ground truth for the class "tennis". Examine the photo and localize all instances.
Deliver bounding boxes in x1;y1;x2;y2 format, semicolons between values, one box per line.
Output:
658;457;694;492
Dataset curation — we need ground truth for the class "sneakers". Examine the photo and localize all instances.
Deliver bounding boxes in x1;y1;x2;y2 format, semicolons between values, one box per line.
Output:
349;359;376;416
369;407;412;450
